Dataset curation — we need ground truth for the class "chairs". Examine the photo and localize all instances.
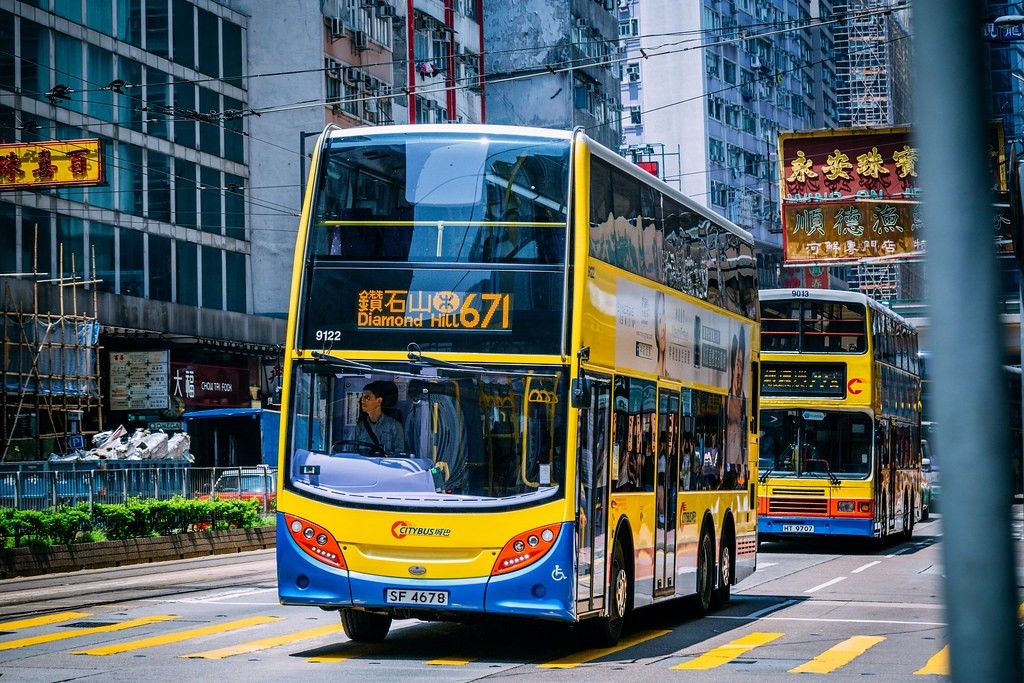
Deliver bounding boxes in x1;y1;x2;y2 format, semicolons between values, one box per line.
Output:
358;381;402;426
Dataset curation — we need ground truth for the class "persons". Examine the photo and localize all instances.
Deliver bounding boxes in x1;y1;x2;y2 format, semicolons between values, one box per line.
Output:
653;290;671;377
343;382;404;458
728;322;746;397
612;395;746;523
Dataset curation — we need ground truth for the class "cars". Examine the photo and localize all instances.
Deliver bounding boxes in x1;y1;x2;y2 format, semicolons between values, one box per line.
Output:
199;469;277;517
920;420;941;514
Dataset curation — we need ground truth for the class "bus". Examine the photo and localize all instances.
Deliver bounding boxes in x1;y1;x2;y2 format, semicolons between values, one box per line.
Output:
259;121;759;647
758;288;923;547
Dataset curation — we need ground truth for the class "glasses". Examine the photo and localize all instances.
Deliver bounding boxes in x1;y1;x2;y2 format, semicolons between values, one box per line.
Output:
361;395;378;402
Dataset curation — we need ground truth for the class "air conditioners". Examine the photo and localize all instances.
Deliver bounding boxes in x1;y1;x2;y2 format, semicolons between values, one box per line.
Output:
586;83;594;92
355;31;368;48
708;82;793;190
632;106;639;112
330;62;338;74
422;19;433;29
380;6;393;16
751;55;762;68
380;86;393;96
348;69;357;79
617;0;628;7
331;18;345;36
627;67;637;74
577;18;588;27
362;0;374;5
441;31;451;41
